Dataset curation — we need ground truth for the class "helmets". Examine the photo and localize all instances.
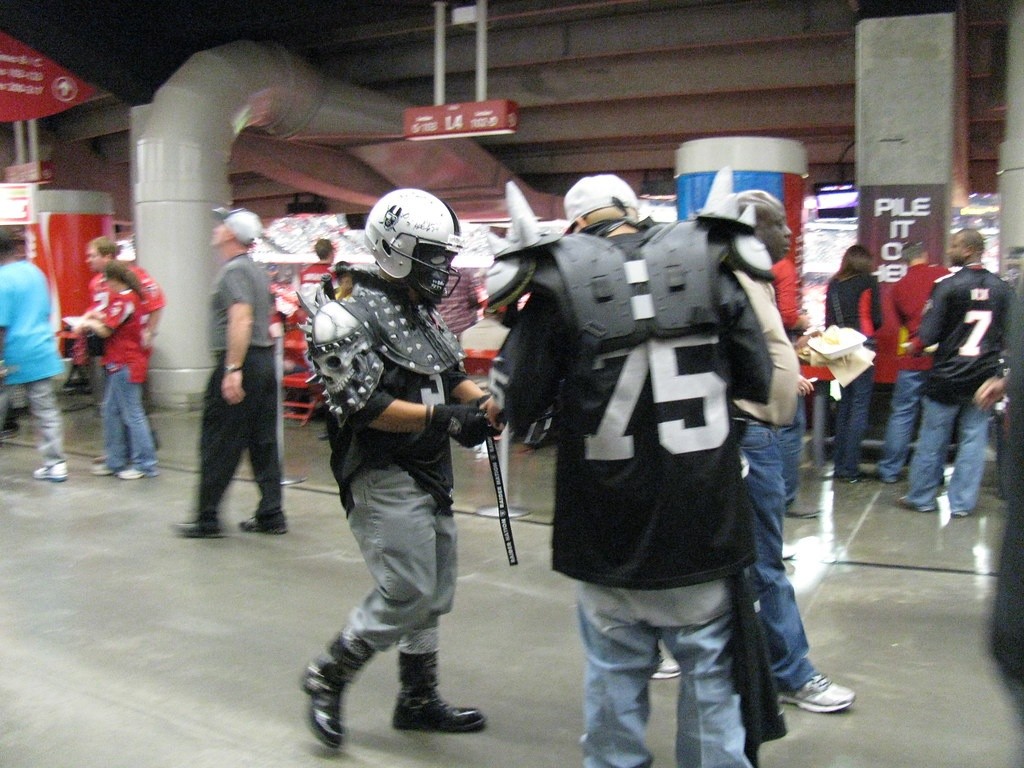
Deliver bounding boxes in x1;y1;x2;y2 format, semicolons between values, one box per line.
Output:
363;189;461;302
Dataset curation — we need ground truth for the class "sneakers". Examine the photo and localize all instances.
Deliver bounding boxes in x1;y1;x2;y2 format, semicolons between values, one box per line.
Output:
650;655;681;679
32;464;69;481
91;462;114;475
242;515;287;534
174;516;221;537
777;673;856;714
118;468;159;479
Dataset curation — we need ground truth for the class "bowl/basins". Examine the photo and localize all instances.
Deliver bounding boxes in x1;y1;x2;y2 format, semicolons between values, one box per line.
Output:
806;327;868;360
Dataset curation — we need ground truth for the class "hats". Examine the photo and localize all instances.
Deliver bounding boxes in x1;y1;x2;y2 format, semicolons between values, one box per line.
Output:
564;175;643;236
212;207;264;246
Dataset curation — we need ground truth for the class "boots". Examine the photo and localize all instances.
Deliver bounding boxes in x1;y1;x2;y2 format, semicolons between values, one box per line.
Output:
301;631;373;749
394;651;485;731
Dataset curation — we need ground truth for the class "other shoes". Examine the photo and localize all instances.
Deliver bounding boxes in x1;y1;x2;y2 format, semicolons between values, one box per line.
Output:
841;473;868;482
787;503;822;520
781;542;795;559
1;422;18;433
823;442;834;460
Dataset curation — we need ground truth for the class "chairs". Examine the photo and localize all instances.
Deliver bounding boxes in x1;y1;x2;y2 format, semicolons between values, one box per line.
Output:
282;329;325;428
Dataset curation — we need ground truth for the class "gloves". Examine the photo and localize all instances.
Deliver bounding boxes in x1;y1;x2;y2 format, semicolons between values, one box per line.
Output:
427;395;503;449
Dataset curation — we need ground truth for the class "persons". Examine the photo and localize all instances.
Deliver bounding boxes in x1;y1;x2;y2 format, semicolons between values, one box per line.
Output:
873;241;952;485
72;236;165;480
298;238;351;440
734;189;824;559
825;245;883;483
484;175;788;768
295;188;508;750
972;246;1024;717
0;228;69;482
897;228;1014;518
167;207;288;538
264;263;292;304
652;190;856;713
434;267;479;342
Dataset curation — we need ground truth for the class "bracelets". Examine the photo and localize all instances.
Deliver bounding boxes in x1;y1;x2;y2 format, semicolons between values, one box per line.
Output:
224;366;242;372
425;405;430;426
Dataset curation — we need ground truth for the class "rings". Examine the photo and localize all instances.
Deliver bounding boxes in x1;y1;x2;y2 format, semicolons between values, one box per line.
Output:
801;387;805;391
